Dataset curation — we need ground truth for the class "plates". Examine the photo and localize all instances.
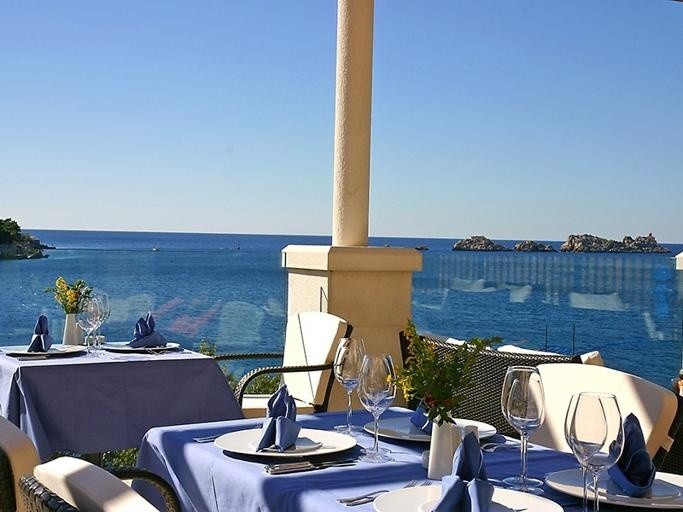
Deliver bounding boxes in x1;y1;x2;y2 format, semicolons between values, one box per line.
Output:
372;485;565;512
100;340;179;353
0;343;86;356
542;465;683;509
212;426;358;459
362;416;497;444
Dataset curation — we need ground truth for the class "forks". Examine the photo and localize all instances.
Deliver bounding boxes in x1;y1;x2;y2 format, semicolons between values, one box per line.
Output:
335;478;432;508
479;443;533;453
145;348;191;355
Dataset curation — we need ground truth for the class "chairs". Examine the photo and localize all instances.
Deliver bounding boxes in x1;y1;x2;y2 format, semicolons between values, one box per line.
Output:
400;328;605;441
212;310;353;418
526;363;681;475
0;414;183;510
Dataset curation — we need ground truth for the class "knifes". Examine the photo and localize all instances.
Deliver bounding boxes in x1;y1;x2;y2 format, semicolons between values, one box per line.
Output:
263;460;356;475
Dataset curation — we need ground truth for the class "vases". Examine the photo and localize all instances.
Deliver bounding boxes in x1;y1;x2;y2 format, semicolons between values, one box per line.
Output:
427;423;454;480
61;313;81;345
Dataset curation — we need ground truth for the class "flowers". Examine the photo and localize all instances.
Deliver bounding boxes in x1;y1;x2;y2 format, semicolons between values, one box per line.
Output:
48;276;92;314
386;316;505;423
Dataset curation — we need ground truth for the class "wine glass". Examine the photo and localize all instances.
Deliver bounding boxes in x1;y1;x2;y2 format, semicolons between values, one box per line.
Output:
332;336;366;433
499;364;545;495
562;390;625;512
75;293;110;357
357;353;396;463
